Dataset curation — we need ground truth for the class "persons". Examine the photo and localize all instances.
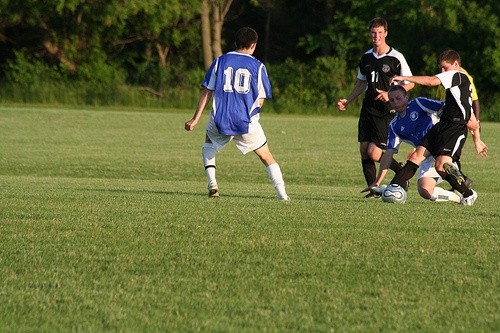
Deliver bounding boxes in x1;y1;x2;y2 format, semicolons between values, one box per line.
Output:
371;50;481;206
185;28;290;202
360;85;488;202
337;18;415;198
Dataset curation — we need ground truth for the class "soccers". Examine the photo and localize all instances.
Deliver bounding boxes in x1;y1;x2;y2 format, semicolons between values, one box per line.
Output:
382;183;407;205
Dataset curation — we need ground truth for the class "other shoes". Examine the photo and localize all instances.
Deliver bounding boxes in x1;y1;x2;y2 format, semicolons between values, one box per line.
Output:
397;161;411;192
208;188;220;197
448;176;473;192
461;188;478;207
363;190;383;200
442;162;466;185
369;183;390;197
